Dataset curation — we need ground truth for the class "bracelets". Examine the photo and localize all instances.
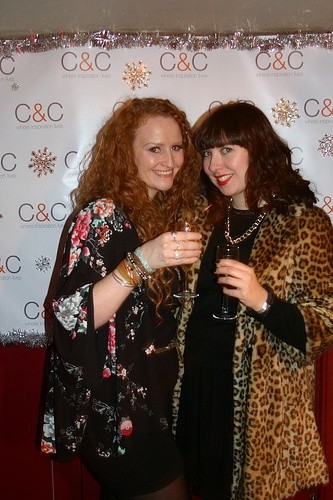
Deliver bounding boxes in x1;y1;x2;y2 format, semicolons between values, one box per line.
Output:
251;282;276;316
111;247;154;290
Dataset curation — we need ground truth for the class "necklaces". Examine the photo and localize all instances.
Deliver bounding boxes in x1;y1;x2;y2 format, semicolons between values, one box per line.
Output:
225;198;270;245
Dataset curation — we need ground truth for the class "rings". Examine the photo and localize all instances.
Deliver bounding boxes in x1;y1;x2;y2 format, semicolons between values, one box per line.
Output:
176;242;181;249
171;231;176;240
174;250;179;260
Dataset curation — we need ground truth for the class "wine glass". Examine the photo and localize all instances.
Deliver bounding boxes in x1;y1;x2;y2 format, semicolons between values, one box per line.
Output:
212;245;240;320
174;222;199;299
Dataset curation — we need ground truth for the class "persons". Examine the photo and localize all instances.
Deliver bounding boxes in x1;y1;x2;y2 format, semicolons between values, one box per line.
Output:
172;102;333;500
39;97;202;500
